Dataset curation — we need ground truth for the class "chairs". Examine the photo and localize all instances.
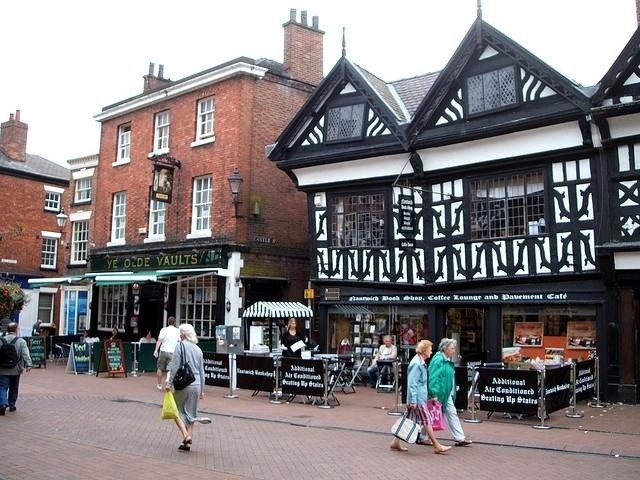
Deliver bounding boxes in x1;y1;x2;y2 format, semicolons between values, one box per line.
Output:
54;331;156;367
251;352;574;421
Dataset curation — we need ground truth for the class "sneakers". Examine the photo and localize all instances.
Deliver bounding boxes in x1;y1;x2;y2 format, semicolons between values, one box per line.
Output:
1;404;17;417
177;434;193;453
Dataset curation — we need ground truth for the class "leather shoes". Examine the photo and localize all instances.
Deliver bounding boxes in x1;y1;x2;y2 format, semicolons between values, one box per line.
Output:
455;438;473;447
417;438;434;446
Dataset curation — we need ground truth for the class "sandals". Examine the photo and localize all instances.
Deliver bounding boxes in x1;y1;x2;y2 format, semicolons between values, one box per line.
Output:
389;445;409;453
434;444;452;454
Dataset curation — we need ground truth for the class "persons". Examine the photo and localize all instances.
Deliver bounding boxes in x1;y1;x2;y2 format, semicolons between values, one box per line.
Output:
32;320;42;337
391;340;452;454
110;328;120;340
281;318;301;358
83;328;100;354
367;335;397;388
83;330;89;338
416;339;472;446
165;323;206;451
138;330;156;350
153;317;178;391
0;322;33;415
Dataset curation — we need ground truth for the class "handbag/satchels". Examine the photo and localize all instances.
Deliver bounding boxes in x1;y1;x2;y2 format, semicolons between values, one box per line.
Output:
172;340;196;392
390;404;421;445
425;398;446;433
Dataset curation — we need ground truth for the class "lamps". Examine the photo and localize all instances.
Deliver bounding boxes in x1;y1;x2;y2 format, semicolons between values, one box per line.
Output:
227;167;246;220
53;206;70;235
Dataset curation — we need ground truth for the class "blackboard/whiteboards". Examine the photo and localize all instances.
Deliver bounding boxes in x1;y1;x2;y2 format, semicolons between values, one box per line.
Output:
72;342;91;372
103;339;126;373
28;336;46;365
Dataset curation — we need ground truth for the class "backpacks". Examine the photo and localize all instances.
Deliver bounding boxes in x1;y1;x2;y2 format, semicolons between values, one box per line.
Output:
1;335;22;370
